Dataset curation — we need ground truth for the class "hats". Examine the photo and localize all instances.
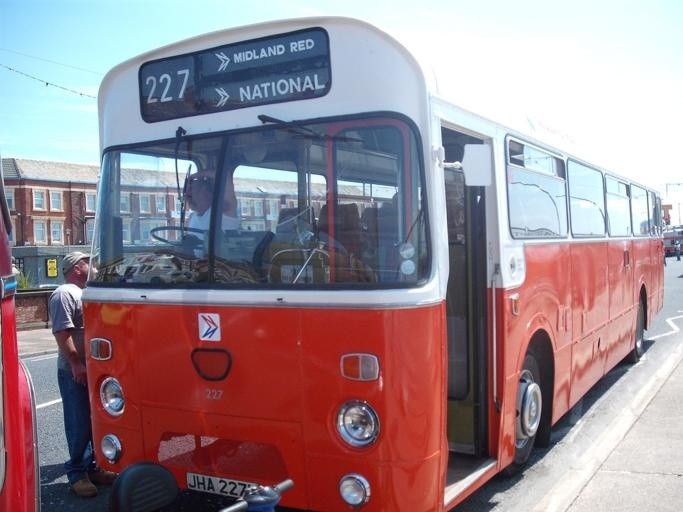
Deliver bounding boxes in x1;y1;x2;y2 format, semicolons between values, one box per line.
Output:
62;251;91;275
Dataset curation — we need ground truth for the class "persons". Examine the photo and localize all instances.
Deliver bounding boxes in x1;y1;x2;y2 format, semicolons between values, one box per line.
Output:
178;171;239;262
46;250;117;501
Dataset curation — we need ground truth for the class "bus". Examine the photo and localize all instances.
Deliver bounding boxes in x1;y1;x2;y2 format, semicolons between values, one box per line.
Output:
2;155;48;512
662;224;683;256
79;18;667;510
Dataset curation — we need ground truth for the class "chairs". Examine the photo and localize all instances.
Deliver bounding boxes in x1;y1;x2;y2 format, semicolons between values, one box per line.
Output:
258;191;399;283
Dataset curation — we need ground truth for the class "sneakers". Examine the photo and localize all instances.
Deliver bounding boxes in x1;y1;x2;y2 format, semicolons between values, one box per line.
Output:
70;479;98;497
88;466;117;483
